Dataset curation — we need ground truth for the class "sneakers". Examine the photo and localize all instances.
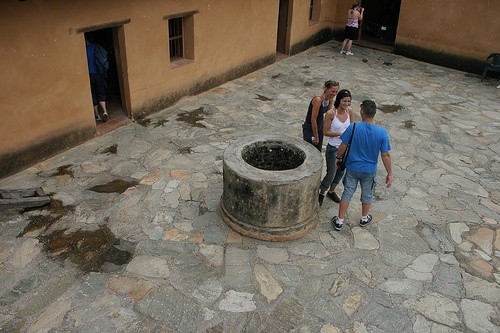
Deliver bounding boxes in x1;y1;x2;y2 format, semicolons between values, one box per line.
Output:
331;215;345;231
95;113;110;121
318;192;324;206
359;214;372;227
338;50;354;56
327;190;341;203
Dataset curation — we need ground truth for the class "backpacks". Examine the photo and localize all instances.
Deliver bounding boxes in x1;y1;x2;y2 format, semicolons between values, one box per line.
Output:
95;46;112;78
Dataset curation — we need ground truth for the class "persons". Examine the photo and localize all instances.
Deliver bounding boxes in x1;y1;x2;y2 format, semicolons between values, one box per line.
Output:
302;80;339;151
340;1;364;56
84;37;108;122
319;89;355;207
332;99;393;231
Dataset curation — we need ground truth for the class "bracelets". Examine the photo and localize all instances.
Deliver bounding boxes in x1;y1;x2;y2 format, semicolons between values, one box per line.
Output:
336;155;342;159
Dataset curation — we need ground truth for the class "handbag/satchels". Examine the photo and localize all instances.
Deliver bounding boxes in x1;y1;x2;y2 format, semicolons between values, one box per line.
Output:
337;160;347;172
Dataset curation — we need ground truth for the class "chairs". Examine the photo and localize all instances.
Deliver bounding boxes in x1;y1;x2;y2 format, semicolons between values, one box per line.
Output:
480;53;500;82
